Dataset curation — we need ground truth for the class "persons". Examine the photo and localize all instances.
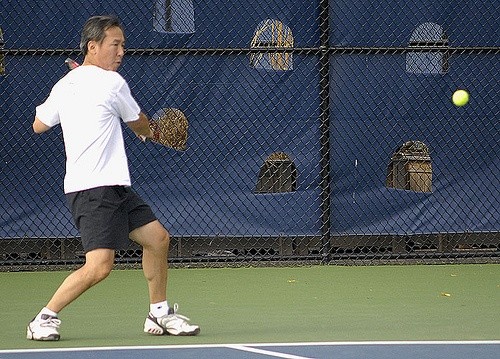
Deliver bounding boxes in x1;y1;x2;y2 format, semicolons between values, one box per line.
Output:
25;15;199;340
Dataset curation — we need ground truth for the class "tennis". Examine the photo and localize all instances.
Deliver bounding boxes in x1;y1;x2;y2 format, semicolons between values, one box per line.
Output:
453;89;469;107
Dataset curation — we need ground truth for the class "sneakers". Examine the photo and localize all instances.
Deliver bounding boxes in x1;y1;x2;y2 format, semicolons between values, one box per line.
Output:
25;312;62;341
143;302;201;336
